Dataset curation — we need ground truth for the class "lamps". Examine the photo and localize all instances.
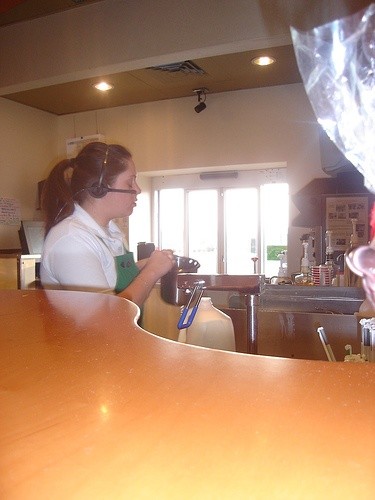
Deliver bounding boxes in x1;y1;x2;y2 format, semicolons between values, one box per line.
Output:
190;87;210;113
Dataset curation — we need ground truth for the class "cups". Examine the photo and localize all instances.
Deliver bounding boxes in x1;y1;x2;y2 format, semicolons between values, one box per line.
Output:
160;256;200;306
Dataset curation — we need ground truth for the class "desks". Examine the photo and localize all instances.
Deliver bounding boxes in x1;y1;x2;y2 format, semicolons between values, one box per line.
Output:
1;286;374;499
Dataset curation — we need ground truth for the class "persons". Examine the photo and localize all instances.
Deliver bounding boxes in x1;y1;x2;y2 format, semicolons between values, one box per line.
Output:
38;141;177;316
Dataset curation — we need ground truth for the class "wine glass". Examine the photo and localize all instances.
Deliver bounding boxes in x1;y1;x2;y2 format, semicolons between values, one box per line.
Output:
288;1;375;190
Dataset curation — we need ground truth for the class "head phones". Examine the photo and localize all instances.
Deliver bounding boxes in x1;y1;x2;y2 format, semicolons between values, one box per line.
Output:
87;144;111;198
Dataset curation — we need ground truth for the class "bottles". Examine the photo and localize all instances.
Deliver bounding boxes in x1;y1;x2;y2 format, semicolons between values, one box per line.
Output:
301;242;311;286
344;218;364;288
319;229;340;288
277;254;284;285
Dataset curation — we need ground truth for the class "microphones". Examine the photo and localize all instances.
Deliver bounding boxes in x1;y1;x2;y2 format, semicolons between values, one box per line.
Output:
104;186;137;194
